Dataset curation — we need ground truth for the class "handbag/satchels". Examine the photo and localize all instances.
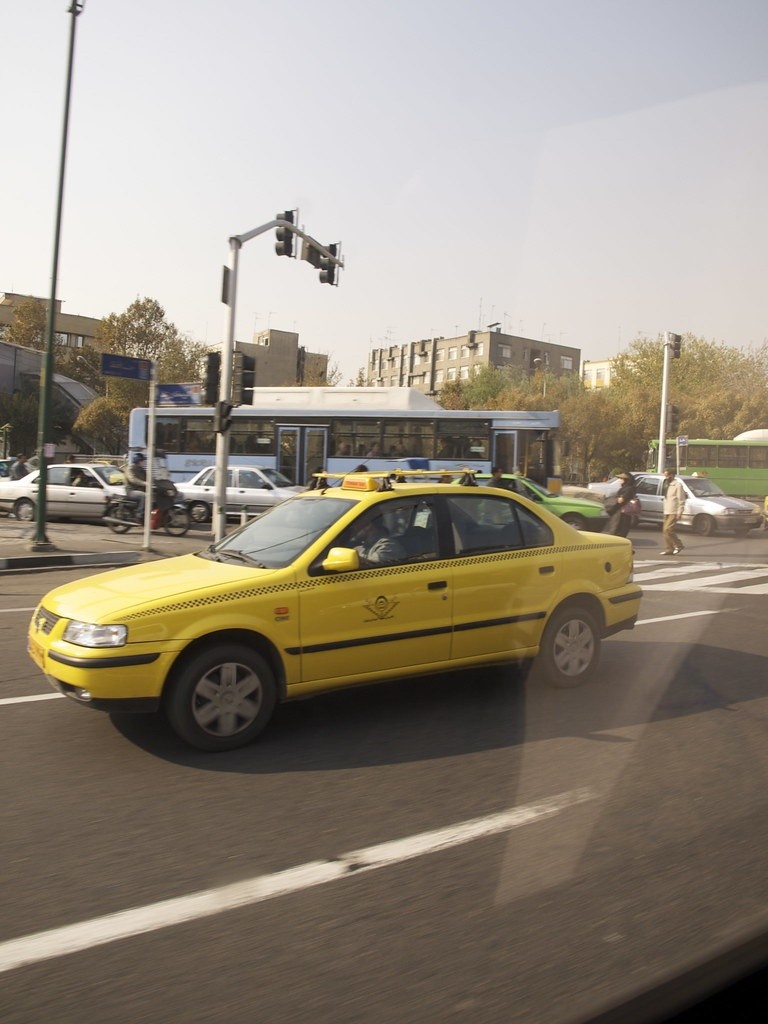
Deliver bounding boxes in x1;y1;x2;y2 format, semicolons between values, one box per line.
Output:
620;499;642;517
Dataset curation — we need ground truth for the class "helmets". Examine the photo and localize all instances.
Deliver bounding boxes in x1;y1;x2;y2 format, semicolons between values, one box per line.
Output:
131;453;147;463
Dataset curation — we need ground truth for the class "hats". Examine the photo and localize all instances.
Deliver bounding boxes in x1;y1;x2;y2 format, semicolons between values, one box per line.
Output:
616;472;633;479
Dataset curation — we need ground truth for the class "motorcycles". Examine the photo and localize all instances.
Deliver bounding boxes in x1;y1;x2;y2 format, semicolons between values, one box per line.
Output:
102;480;193;538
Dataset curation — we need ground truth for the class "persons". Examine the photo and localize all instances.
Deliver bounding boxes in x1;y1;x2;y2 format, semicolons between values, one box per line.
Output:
71;470;88;487
486;466;526;492
335;427;483;458
309;467;332;490
659;468;686;556
146;422;259;455
9;453;29;481
124;453;157;524
697;471;708;478
338;465;368;486
63;454;76;465
437;470;453;483
352;506;407;566
604;472;636;556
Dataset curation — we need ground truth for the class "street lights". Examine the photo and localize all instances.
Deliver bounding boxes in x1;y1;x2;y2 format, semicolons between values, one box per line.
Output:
32;0;88;552
76;356;109;398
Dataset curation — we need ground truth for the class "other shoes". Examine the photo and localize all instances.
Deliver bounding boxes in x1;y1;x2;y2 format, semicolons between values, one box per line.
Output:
660;546;683;556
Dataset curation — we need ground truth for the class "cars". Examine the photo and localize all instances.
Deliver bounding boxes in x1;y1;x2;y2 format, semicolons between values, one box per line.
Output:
447;472;613;532
610;475;765;538
168;464;306;522
30;477;643;757
0;459;15;481
587;472;646;494
0;462;130;522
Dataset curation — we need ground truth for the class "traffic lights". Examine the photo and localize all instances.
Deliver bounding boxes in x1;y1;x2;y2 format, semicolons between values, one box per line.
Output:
213;402;232;432
672;333;681;359
274;210;292;257
319;243;337;284
232;353;255;405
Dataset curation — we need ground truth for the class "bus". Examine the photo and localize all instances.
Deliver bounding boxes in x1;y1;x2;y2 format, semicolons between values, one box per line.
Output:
129;388;570;490
644;439;768;498
